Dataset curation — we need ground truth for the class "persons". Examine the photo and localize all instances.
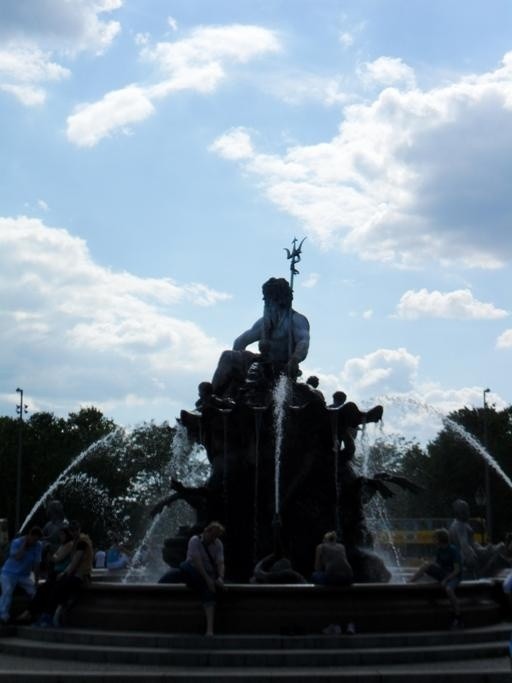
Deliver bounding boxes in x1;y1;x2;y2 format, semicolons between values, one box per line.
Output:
407;529;464;631
315;531;356;634
0;500;162;628
449;499;512;578
195;277;310;413
179;522;225;640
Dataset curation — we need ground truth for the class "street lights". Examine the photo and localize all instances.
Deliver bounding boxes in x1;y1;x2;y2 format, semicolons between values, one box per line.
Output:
482;388;497;542
13;388;27;532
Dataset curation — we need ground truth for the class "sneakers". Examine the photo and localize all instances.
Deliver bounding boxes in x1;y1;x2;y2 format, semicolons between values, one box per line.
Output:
321;622;342;636
346;623;357;635
449;619;465;632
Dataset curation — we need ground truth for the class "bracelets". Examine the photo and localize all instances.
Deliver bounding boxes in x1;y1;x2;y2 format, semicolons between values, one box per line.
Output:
217;574;225;581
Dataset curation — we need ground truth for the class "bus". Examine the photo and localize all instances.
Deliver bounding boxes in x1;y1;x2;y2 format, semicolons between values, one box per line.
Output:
373;516;491;567
